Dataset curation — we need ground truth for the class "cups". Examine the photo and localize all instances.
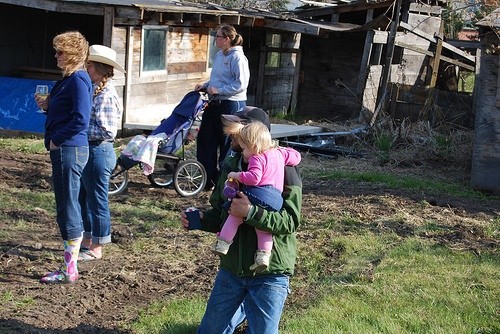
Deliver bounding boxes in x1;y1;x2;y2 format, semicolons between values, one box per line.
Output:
184;210;203;230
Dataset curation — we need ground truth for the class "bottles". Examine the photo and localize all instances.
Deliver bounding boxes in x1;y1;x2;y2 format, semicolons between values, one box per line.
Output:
222;177;240;199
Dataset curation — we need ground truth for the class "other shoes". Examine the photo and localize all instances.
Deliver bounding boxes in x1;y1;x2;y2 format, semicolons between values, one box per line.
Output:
249;251;270;273
78;244;100;260
210;238;234;255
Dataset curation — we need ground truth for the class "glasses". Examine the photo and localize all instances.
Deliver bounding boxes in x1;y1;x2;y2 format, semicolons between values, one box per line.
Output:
57;51;64;57
216;34;225;38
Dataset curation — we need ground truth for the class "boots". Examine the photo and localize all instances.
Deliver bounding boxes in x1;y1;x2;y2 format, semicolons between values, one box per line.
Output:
40;238;82;285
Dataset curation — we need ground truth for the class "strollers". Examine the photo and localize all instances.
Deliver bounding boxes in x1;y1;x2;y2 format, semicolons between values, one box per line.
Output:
107;87;215;197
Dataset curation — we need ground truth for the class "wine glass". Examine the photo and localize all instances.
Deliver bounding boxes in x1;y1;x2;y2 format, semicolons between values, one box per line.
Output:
36;85;49;113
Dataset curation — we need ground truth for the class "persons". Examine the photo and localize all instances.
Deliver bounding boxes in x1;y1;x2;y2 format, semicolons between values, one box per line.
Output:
192;24;250;191
180;106;303;334
32;32;93;284
76;45;126;261
209;121;303;272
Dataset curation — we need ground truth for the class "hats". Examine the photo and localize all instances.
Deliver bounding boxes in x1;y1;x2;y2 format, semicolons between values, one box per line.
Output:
88;44;126;74
221;105;271;133
